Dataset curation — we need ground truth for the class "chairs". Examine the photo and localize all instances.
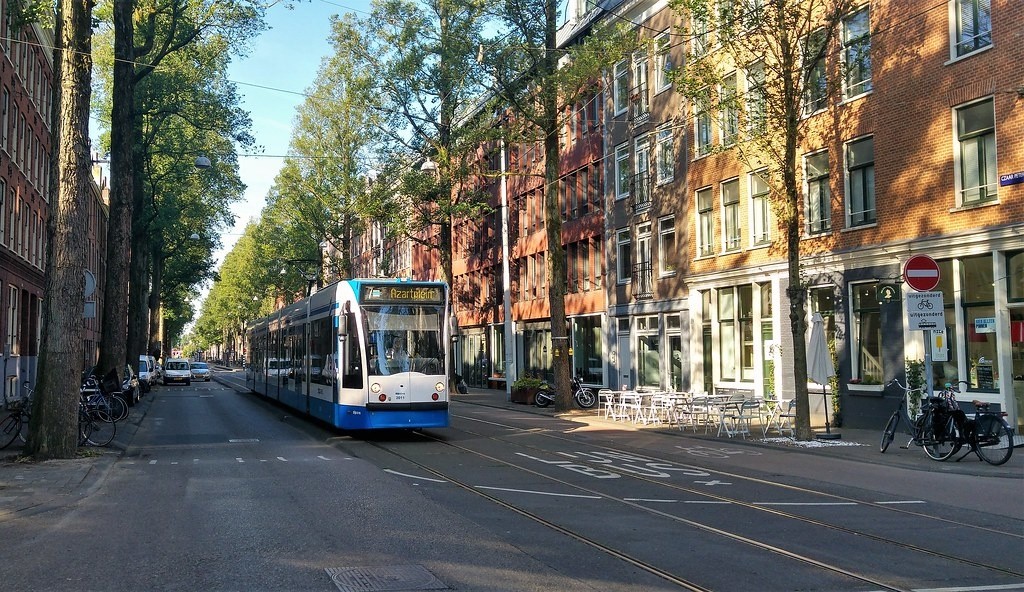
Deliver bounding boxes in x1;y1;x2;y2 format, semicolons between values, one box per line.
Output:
598;388;796;441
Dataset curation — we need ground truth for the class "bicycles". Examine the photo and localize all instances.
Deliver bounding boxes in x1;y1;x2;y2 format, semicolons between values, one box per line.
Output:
879;378;1015;465
0;372;129;451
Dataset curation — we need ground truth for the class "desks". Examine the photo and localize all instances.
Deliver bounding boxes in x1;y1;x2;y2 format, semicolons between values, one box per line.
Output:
653;396;688;431
626;393;655;424
698;394;732;437
762;400;791;436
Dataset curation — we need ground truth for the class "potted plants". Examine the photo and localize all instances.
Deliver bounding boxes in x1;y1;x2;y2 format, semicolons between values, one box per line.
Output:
511;377;545;405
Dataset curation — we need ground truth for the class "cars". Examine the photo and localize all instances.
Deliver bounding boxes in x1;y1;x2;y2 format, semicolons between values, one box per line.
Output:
88;364;140;407
190;362;211;382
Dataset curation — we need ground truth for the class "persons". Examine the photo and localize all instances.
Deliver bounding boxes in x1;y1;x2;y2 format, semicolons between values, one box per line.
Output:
386;338;407;359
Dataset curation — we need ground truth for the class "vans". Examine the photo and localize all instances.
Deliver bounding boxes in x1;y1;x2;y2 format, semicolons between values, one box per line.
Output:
139;354;162;393
303;354;325;382
321;354;338;387
263;357;302;380
162;358;193;386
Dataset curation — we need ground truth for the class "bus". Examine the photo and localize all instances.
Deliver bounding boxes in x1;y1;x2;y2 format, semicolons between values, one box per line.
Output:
246;259;459;429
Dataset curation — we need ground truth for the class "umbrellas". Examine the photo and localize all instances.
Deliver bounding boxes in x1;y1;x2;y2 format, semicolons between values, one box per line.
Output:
807;311;841;439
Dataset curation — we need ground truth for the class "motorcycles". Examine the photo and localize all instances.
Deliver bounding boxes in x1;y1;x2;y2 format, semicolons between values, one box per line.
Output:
534;374;596;408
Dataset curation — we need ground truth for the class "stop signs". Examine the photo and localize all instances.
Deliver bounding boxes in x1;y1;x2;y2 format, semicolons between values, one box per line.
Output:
903;254;940;292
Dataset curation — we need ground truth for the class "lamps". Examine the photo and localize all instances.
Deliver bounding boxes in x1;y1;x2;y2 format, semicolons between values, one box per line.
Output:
194;155;211;168
420;157;436;171
190;232;200;240
